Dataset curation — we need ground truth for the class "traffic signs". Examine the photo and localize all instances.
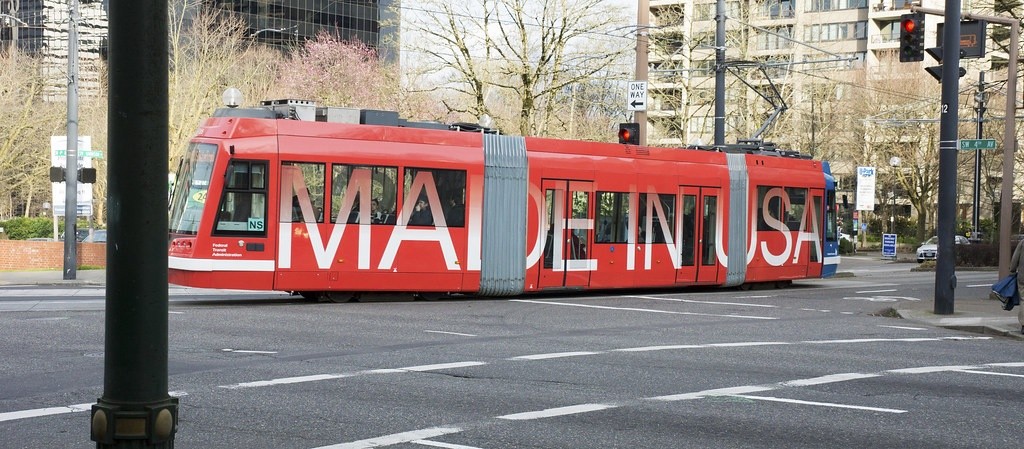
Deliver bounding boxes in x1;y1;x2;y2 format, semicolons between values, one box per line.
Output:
627;80;647;112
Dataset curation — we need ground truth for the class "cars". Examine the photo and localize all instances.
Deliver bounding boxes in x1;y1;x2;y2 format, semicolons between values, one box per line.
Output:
916;234;973;264
27;226;107;244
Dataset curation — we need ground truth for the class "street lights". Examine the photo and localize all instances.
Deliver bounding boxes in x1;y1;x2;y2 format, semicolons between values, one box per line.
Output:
891;157;900;259
42;202;51;220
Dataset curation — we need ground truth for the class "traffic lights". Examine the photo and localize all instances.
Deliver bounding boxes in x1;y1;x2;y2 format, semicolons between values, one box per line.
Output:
899;12;925;63
617;121;641;146
925;44;967;84
76;167;97;184
49;166;63;183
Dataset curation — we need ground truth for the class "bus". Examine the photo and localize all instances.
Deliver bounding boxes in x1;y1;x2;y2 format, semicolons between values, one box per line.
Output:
167;55;841;303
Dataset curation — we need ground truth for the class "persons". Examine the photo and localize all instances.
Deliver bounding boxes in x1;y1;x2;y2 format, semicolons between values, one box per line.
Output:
960;221;973;241
292;187;790;267
1007;239;1024;334
